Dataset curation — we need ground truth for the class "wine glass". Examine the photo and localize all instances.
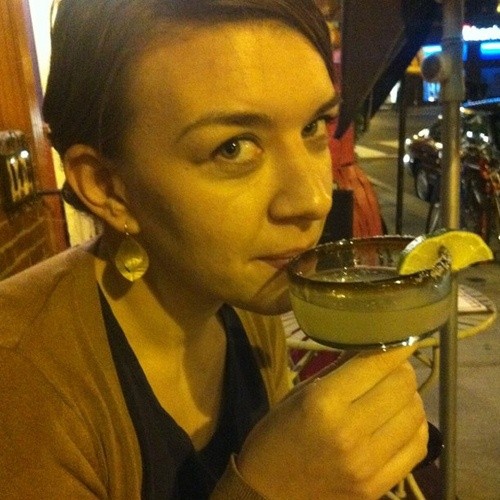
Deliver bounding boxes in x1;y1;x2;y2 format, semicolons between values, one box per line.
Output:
287;233;444;470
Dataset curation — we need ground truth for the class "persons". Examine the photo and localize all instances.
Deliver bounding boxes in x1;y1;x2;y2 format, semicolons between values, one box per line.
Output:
0;0;433;500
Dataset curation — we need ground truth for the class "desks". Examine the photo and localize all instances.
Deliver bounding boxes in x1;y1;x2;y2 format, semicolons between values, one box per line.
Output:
279;282;497;500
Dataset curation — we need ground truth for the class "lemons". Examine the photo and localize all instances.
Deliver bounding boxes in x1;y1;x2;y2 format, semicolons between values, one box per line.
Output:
394;227;495;276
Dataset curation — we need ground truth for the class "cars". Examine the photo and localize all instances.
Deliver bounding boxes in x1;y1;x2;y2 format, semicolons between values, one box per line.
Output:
403;97;500;234
